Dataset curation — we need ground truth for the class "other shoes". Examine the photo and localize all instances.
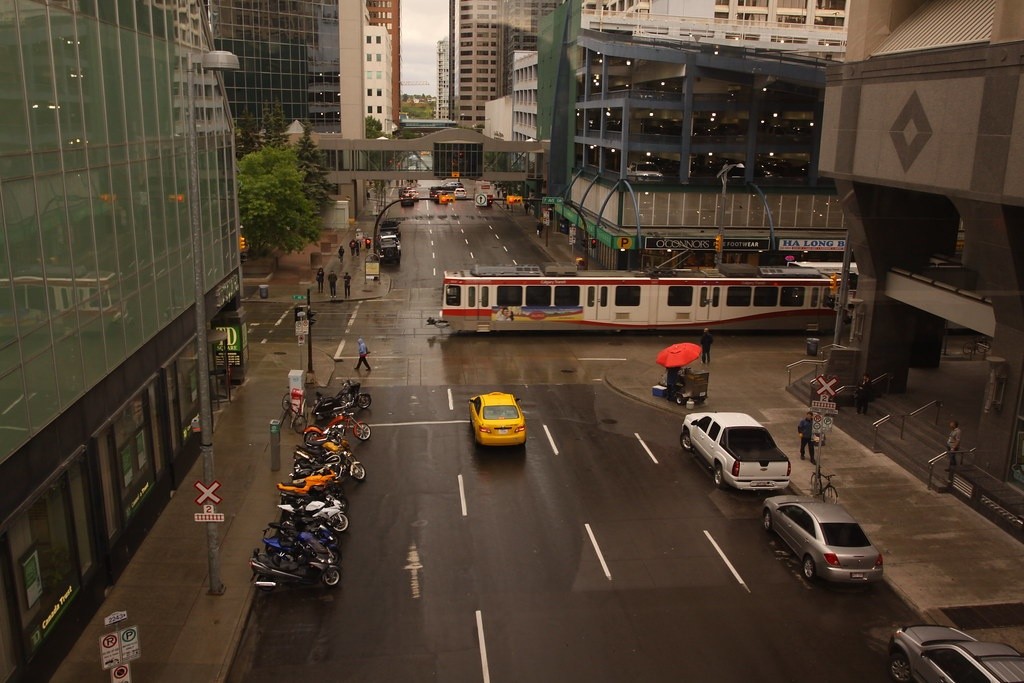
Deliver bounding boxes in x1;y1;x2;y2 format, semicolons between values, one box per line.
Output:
801;455;804;459
811;458;816;464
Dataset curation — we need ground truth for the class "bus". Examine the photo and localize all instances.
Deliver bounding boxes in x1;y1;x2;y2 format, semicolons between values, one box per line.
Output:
440;249;849;335
789;259;858;324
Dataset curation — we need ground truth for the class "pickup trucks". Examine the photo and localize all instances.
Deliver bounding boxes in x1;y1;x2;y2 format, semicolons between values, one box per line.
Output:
679;411;792;493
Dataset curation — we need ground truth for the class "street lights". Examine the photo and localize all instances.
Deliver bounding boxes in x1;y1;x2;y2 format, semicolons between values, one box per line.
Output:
715;161;746;263
185;49;241;596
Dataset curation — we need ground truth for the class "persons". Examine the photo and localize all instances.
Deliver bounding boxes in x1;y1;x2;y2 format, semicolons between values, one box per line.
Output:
944;421;961;471
349;235;365;256
497;306;512;321
344;273;350;295
295;306;303;321
354;339;370;370
338;246;344;262
537;221;543;237
701;328;713;363
855;375;871;415
797;412;816;465
317;268;324;293
328;271;337;297
524;203;529;214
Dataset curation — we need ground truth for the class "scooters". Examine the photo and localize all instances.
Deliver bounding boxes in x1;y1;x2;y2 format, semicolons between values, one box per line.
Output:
247;377;375;591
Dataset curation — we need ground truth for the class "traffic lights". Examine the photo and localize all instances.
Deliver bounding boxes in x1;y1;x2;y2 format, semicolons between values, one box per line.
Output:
830;274;838;292
588;238;597;249
715;235;722;253
365;238;372;251
439;195;456;205
507;195;523;205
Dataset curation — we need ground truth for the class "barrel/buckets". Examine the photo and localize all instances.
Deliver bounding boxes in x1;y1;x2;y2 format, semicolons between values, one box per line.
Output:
686;401;694;409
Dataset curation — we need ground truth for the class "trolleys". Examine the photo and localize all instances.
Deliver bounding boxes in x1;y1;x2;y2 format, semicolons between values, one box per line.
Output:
658;366;710;406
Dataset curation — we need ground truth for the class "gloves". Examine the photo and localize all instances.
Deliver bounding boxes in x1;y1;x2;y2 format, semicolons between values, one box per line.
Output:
799;433;802;437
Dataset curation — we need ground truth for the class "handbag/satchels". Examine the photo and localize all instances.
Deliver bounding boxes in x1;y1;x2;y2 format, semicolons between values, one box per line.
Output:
853;390;860;398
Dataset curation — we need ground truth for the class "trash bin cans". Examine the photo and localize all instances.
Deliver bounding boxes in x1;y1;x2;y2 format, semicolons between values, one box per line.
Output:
806;338;820;356
258;285;269;299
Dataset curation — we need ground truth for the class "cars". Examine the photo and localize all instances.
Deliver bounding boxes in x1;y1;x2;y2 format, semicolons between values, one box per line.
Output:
887;625;1024;683
625;160;665;186
763;493;885;585
468;391;527;447
378;219;402;233
397;181;494;207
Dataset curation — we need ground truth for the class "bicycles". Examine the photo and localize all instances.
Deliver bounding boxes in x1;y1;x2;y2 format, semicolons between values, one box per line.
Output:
810;464;839;505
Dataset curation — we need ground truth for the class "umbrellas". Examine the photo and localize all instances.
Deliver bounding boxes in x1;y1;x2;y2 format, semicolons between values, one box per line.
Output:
656;343;702;368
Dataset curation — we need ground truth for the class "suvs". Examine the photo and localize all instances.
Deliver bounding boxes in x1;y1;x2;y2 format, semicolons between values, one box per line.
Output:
377;231;404;264
